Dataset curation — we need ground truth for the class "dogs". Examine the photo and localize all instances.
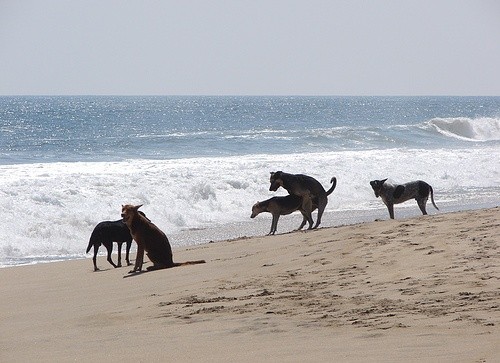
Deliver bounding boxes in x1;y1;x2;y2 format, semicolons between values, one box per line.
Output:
250;177;337;236
269;170;326;229
120;203;206;273
369;178;440;219
85;211;151;272
